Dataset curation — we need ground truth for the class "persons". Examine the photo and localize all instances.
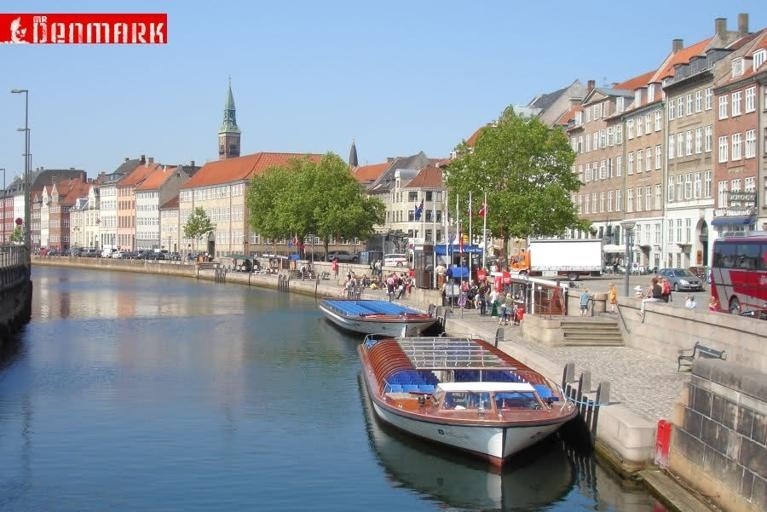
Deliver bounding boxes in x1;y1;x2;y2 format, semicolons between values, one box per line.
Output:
639;276;661;323
579;287;594;318
604;282;617;313
685;294;695;310
706;295;720;312
659;275;672;303
343;258;525;327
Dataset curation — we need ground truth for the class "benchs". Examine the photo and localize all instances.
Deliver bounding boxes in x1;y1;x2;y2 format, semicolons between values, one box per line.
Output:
678;341;725;373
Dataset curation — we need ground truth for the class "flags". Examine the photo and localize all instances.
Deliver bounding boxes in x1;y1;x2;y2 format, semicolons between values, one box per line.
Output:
415;197;423;220
478;195;488;217
467;198;471;216
429;205;434;221
415;204;419;222
459;232;464;256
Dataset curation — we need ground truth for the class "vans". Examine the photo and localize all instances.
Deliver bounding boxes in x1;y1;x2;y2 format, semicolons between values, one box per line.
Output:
385;254;408;268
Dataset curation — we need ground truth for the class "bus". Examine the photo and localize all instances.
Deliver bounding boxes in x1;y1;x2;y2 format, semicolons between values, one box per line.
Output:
707;235;767;320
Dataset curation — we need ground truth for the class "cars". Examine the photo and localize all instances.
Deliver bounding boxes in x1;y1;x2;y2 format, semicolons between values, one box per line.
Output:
328;250;358;262
656;268;702;292
32;249;181;260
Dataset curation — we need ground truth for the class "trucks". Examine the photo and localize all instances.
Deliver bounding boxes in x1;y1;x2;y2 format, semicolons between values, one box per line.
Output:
509;239;604;281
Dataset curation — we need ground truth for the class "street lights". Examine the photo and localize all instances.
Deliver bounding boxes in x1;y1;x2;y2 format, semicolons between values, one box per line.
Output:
312;236;319;268
0;87;32;244
621;221;636;296
